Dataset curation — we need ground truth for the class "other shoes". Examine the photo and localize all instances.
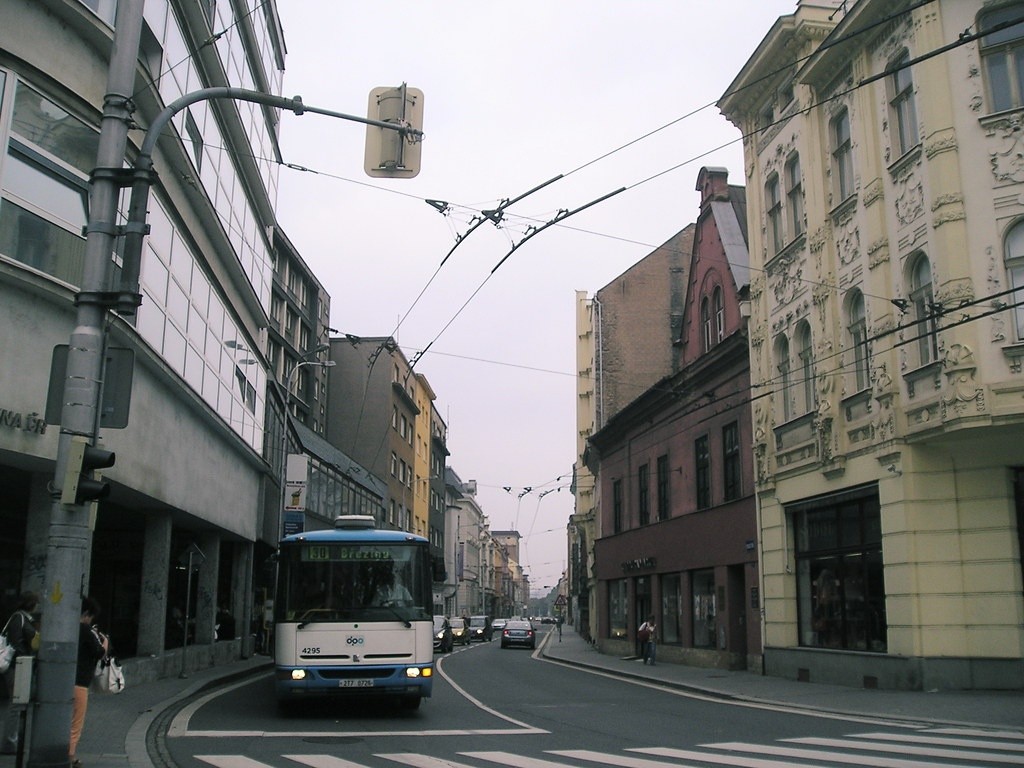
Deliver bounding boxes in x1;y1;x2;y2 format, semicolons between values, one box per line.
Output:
67;759;81;768
650;662;656;666
644;659;647;664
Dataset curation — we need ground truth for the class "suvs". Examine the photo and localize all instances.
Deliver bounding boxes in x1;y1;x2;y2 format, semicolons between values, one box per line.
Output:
469;616;493;643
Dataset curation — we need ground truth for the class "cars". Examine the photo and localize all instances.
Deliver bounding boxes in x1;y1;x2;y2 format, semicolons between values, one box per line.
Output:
432;614;454;653
529;616;558;624
449;618;471;646
500;620;537;650
491;616;528;630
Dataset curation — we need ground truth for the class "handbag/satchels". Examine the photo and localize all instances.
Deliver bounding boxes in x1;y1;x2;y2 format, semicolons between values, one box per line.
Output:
91;626;125;694
0;612;23;673
639;622;649;641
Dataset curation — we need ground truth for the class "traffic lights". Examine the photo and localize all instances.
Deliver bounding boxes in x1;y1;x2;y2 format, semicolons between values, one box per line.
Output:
61;437;115;506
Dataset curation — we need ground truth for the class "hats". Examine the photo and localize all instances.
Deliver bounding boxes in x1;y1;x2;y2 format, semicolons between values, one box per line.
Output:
19;589;39;605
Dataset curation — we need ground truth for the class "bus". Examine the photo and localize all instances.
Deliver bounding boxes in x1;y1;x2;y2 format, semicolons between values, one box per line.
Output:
266;515;446;712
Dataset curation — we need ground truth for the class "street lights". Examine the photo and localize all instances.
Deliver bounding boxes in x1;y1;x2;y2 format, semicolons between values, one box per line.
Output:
401;476;440;529
271;341;339;611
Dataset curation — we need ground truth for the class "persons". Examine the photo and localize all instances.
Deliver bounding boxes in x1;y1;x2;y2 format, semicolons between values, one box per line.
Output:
371;564;412;606
253;600;264;656
68;598;109;768
0;594;40;755
639;614;657;664
